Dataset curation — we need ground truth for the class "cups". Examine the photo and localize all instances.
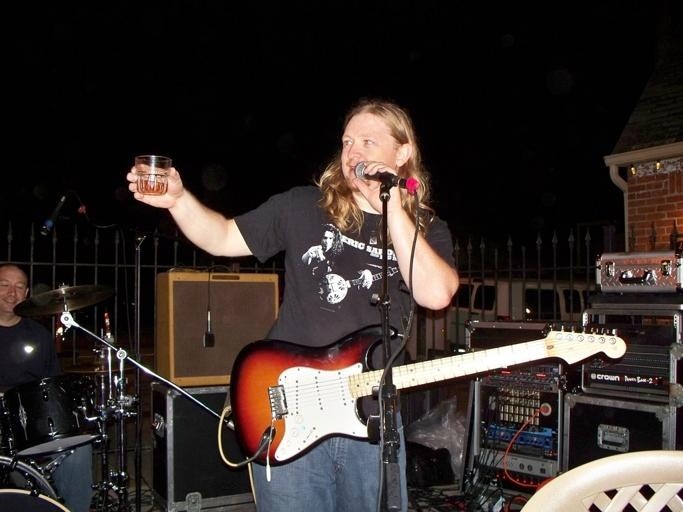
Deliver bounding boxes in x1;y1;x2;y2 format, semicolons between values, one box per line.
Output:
135;155;169;195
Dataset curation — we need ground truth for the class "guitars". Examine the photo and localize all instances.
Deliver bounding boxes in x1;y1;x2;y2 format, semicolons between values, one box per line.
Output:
230;324;627;466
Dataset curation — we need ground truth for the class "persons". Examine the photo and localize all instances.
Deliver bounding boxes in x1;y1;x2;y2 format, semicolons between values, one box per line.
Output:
1;265;56;391
302;222;373;310
125;101;461;512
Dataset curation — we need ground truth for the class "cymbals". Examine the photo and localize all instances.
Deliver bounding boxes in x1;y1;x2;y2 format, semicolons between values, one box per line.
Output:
13;285;112;317
64;361;137;373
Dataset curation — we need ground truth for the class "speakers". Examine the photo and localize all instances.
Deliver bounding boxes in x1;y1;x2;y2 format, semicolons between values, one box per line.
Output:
154;271;280;386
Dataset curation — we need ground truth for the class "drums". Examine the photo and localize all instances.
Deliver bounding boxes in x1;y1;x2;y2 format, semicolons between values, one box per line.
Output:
0;372;103;456
0;457;71;511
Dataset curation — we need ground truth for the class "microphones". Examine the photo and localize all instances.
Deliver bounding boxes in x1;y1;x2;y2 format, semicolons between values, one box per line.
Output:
38;193;69;238
353;161;420;194
202;308;216;348
